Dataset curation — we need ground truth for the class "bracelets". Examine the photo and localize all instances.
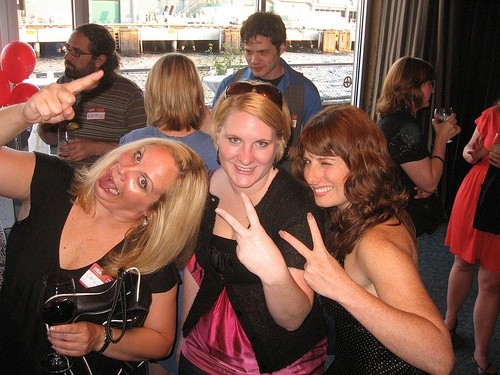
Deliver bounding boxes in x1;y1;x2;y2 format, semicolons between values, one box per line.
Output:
97;326;112;353
432;156;445;168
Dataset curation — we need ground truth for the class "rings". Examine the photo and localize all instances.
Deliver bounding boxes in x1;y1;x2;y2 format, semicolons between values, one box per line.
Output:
456;123;457;125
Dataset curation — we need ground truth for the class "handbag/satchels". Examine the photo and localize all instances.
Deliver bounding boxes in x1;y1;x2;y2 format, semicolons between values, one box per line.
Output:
35;262;153;375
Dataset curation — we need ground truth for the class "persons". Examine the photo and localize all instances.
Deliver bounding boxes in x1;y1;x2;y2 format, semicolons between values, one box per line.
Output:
0;70;210;375
36;23;148;166
175;81;329;375
116;53;221;375
214;11;323;133
277;104;455;375
443;104;500;375
374;56;461;214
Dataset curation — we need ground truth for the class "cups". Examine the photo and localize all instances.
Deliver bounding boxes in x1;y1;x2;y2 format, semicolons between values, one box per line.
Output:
491;133;500;153
58;128;74;160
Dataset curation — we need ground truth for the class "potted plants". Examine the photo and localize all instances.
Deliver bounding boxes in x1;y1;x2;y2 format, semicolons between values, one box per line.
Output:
201;42;245;95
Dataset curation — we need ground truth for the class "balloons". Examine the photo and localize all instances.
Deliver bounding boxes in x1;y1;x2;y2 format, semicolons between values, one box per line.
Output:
0;72;12;107
0;41;36;84
10;83;40;105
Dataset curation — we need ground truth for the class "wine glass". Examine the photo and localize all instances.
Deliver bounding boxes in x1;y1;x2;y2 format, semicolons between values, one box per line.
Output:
434;108;453;143
39;271;77;373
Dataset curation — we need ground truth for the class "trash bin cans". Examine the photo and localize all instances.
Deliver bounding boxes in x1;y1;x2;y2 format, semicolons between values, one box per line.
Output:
338;31;351;53
320;31;337;53
223;28;239;51
119;28;141;57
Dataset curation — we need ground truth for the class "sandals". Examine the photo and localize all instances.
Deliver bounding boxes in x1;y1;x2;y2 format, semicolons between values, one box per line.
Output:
472;354;497;375
443;316;458;349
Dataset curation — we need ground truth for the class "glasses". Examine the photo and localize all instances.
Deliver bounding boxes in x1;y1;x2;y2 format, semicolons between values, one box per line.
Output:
225;82;283;112
60;43;92;58
